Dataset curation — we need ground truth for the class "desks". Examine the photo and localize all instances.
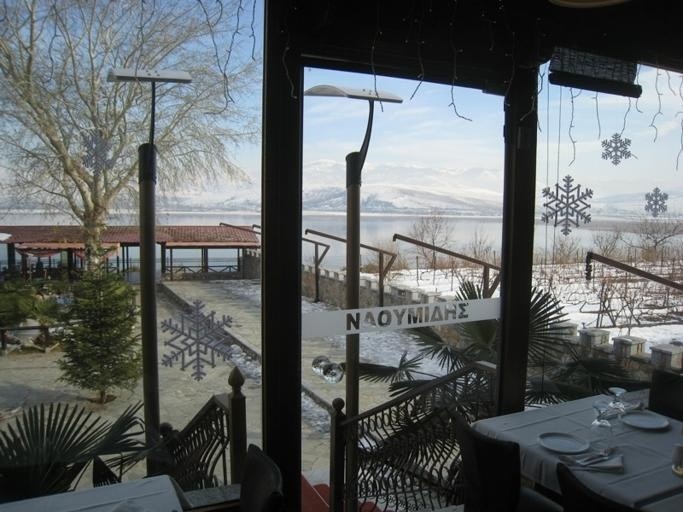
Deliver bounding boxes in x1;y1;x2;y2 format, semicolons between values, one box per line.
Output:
0;476;184;511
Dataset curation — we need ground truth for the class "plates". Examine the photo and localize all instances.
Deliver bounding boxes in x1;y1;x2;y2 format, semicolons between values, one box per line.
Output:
618;411;669;430
536;430;590;453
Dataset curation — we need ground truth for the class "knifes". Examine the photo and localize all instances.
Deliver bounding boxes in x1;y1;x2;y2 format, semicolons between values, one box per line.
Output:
580;450;622;468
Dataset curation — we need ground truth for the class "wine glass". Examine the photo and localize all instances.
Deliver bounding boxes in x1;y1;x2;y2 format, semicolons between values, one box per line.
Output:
604;386;626;426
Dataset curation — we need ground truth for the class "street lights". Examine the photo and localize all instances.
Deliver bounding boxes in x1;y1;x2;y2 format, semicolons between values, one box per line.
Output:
302;85;404;511
105;67;193;478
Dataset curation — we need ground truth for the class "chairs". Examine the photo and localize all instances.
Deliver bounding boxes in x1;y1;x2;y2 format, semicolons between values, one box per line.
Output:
185;443;282;512
449;367;683;512
